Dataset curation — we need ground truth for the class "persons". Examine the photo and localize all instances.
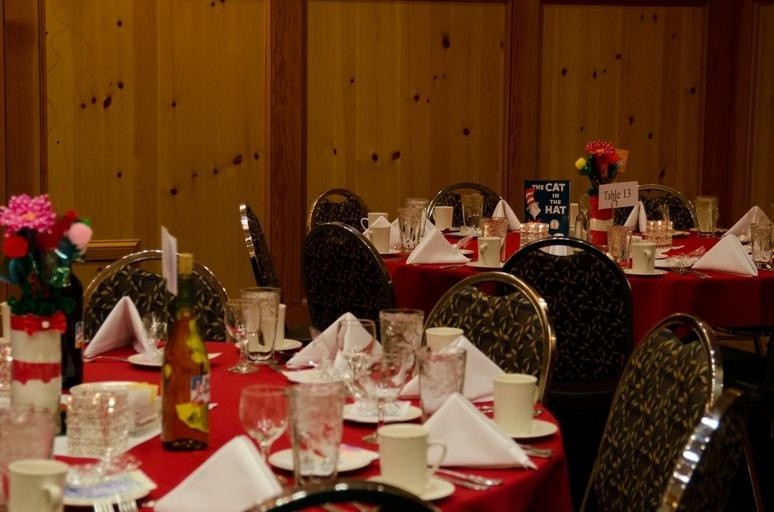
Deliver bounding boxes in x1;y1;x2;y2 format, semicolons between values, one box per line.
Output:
192;379;203;402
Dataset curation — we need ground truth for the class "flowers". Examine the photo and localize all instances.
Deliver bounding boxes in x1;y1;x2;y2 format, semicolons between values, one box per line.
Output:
577;138;626;196
5;193;99;263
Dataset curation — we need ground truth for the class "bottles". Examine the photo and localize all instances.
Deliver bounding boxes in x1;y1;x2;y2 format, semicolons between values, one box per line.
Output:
572;193;591;242
592;197;614;248
57;254;85;433
162;258;210;448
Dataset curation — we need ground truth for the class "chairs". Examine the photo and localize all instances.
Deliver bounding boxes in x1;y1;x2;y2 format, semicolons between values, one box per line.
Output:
500;237;634;491
708;330;771;473
610;184;702;231
309;188;373;236
576;313;724;511
424;183;503;225
82;249;237;345
300;222;399;348
420;271;556;406
240;204;310;345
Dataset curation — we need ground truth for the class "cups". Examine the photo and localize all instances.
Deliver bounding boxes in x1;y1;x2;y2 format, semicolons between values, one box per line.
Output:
287;381;344;488
273;301;285;348
691;196;717;237
749;220;772;273
377;305;426;376
646;219;673;253
366;224;390;252
606;223;631;263
425;326;463;353
485;217;509;262
1;377;159;512
0;337;12;397
432;206;453;233
376;423;448;490
492;374;542;435
358;211;392;226
459;193;483;234
416;345;469;419
477;237;502;264
238;285;281;368
631;241;655;271
397;197;429;251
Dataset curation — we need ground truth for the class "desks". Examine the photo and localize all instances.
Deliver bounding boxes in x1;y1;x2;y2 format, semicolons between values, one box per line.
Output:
2;337;572;510
368;227;772;355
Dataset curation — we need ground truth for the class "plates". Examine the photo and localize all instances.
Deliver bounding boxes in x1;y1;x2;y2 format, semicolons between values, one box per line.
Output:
341;401;424;425
367;475;457;504
270;443;370;476
373;246;401;256
126;352;162;367
465;260;510;273
488;418;558;438
622;268;670;277
234;338;304;353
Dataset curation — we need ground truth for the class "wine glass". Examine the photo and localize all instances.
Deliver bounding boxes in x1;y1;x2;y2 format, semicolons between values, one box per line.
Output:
333;317;376;401
342;349;412;444
224;299;262;375
237;384;292;465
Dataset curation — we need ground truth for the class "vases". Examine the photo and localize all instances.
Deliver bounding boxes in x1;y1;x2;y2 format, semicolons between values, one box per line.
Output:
7;312;65;437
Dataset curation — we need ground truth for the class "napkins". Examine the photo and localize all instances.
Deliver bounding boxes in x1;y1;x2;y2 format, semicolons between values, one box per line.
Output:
624;199;647;233
85;295;155;356
421;392;537;470
155;433;285;512
402;334;508;405
405;226;471;264
491;196;523;230
286;314;384;369
691;231;758;275
363;216;404;243
